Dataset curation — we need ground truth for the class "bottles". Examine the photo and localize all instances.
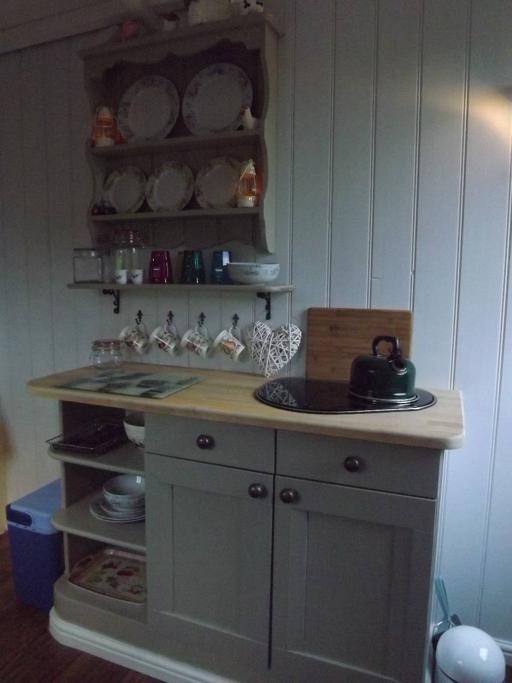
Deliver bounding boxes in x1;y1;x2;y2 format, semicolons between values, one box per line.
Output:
85;336;126;370
91;103;115;148
107;229;151;284
70;247;104;283
237;160;259;207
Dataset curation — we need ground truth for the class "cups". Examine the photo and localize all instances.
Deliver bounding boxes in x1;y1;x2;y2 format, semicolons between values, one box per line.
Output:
213;326;249;364
147;249;175;283
150;318;183;358
209;250;236;285
181;322;216;362
180;250;210;285
118;320;150;354
114;269;144;285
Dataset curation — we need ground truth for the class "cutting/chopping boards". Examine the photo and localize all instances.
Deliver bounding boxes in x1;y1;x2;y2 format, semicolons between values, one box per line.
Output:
306;307;413;381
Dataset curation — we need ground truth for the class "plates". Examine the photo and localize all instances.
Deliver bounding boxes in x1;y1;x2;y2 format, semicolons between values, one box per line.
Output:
182;62;253;135
143;159;195;211
86;492;145;525
102;164;147;213
186;0;243;27
114;75;181;146
194;155;243;209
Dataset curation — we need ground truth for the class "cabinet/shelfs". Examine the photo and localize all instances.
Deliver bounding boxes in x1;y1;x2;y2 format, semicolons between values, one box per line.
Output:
77;12;286;255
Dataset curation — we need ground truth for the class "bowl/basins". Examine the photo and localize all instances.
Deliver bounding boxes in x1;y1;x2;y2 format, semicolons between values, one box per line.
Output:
226;260;280;287
102;476;146;512
122;412;145;449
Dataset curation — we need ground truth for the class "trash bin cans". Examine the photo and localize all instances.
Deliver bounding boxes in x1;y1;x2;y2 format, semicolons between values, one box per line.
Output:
435;625;506;683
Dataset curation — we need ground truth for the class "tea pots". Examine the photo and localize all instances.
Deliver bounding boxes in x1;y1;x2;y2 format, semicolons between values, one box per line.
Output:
345;334;419;405
